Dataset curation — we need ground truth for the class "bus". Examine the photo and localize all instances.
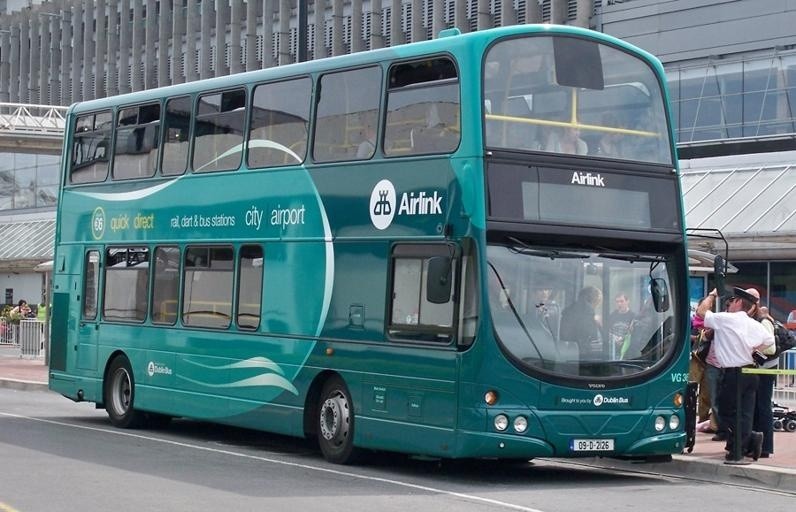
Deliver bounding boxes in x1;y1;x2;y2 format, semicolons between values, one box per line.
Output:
48;22;729;466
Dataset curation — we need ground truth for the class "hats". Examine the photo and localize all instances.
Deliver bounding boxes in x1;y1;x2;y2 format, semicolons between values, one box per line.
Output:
760;307;768;316
733;287;760;303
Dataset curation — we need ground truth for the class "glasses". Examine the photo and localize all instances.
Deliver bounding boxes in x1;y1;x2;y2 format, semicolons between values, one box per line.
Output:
729;297;735;302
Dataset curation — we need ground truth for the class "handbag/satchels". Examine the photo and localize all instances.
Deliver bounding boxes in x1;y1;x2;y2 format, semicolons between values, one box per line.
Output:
752;317;796;366
692;341;711;368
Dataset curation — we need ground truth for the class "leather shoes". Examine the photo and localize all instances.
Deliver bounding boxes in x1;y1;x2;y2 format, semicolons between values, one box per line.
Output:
751;431;763;461
712;431;729;441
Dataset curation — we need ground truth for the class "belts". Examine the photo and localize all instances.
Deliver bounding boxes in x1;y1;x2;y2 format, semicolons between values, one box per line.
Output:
725;367;741;372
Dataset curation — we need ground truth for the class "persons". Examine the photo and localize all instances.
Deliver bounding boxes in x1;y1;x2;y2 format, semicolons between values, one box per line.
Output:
13;300;26;313
357;123;399;160
562;285;602;343
515;111;636;161
609;292;635;359
535;289;561;342
691;286;796;462
